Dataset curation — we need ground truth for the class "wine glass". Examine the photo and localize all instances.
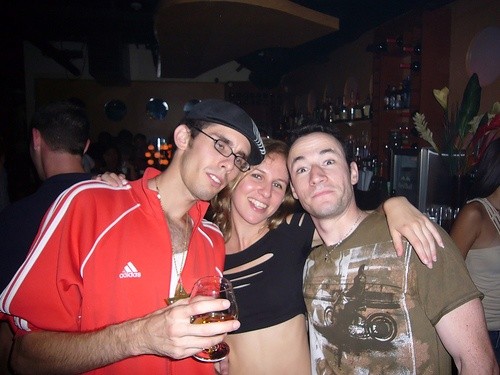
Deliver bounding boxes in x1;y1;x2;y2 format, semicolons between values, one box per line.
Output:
191;275;240;362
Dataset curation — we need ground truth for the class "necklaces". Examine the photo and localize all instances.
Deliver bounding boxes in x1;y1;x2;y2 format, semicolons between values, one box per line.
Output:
154;177;189;256
322;209;365;263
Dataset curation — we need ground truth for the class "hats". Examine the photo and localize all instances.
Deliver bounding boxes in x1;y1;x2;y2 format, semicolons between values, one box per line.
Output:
186;98;266;167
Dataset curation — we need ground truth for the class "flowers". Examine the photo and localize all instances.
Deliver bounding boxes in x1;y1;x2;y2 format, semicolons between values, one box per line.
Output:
412;73;500;185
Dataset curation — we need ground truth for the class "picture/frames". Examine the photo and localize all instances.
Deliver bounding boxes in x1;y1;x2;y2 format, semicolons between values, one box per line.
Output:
390;150;418;209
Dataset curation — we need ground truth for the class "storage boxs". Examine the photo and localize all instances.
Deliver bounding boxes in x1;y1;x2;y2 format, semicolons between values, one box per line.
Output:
123;44;157;80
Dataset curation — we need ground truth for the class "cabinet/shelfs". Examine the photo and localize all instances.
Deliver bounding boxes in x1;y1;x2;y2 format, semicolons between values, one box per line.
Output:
373;5;431;171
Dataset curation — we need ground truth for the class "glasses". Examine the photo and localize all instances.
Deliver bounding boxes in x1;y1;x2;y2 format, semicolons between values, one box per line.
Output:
194;128;250;174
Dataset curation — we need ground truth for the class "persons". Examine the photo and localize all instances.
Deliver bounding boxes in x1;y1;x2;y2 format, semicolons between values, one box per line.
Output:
0;96;500;374
450;138;500;367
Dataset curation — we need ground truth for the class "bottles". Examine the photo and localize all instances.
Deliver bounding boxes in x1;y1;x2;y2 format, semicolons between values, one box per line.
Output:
376;163;387;195
383;76;410;110
423;206;460;230
365;36;402;52
277;92;378;199
400;45;423;73
383;127;420;154
